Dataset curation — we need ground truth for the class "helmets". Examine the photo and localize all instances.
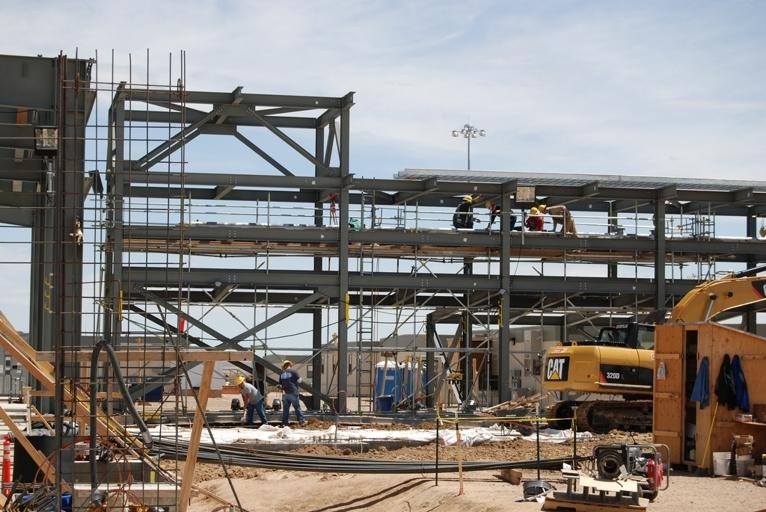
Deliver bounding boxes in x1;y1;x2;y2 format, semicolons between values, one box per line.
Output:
283;360;293;366
235;376;245;385
463;196;473;202
531;204;547;214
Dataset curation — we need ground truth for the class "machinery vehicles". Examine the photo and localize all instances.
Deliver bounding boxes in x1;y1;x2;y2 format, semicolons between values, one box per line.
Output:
542;263;765;471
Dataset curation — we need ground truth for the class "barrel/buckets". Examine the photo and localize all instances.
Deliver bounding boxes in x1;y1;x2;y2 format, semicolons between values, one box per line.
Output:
735;454;753;477
712;452;732;477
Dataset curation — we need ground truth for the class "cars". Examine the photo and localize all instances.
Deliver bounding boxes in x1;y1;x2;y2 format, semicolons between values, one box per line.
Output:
23;420;80;436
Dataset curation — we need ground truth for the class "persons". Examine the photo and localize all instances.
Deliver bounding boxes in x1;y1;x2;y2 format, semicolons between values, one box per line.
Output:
453;195;480;229
279;360;308;428
235;376;268;425
526;207;544;231
485;200;522;231
538;204;577;233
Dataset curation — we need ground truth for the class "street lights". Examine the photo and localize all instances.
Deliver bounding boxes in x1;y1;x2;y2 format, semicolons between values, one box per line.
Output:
451;124;487;169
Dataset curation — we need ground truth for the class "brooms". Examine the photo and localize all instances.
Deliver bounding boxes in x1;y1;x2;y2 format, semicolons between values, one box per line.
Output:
693;400;718;474
